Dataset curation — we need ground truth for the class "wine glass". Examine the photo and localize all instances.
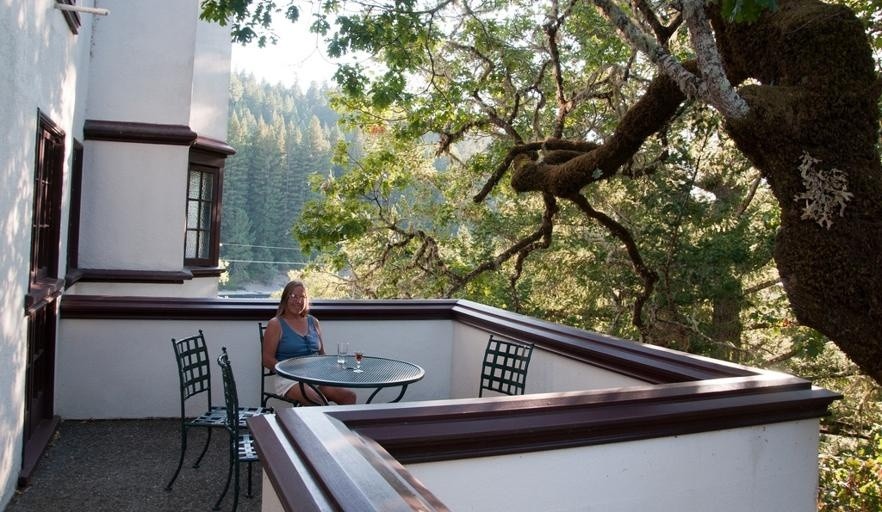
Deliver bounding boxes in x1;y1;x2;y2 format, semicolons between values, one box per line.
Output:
353;352;364;374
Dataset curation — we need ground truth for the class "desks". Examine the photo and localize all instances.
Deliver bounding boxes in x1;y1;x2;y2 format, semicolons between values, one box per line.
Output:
274;355;425;405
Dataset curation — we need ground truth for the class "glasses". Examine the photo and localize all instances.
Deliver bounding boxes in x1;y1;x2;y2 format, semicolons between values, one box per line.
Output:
289;293;306;300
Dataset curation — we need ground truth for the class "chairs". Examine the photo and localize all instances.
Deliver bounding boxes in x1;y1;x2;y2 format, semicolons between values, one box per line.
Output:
259;323;298;407
164;329;273;491
212;347;260;512
478;335;534;397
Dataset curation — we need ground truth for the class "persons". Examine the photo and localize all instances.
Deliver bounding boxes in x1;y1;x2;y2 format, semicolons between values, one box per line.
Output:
261;280;358;406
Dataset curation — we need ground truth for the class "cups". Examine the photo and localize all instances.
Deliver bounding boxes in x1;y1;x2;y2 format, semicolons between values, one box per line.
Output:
337;342;349;364
